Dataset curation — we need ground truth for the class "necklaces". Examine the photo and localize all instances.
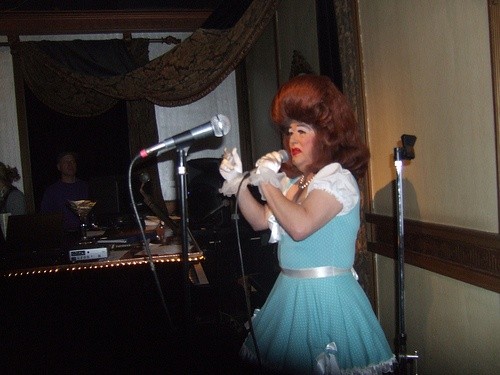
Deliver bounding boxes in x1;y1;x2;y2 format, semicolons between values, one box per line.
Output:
298;175;315;189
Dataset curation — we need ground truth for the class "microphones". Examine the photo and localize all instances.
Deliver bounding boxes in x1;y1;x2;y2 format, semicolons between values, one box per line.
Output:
244;149;290;180
138;114;231;157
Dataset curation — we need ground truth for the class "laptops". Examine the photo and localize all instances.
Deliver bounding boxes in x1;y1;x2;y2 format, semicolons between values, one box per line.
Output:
5;212;65;251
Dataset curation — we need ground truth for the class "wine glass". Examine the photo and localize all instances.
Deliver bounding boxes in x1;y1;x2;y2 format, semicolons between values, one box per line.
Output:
65;198;99;246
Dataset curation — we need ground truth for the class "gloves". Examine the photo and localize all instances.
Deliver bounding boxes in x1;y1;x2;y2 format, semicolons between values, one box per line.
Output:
218;147;248;198
248;151;285;200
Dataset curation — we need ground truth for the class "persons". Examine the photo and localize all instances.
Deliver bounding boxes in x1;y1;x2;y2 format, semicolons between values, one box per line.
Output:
219;73;397;374
186;156;234;325
40;149;88;214
0;161;26;214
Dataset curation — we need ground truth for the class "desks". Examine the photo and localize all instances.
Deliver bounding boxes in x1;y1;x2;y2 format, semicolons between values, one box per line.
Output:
0;216;204;280
